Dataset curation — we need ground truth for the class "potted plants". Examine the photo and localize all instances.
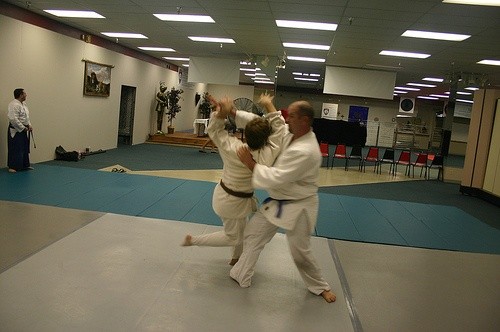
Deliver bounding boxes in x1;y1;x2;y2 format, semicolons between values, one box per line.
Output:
165;87;184;135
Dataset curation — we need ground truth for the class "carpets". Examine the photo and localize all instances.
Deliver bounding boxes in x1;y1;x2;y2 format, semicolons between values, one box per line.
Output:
0;139;500;254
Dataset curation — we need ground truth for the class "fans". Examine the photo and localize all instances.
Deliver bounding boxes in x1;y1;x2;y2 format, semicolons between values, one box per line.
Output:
228;98;258;142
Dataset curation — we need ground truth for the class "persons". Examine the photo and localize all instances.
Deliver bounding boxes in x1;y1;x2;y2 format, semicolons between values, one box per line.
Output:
8;88;35;172
182;93;285;265
208;95;336;303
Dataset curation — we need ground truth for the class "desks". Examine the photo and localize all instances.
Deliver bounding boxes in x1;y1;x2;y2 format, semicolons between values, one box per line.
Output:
193;119;209;138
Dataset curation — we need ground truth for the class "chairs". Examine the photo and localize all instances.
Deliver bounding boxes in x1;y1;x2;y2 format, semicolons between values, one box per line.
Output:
319;143;444;182
